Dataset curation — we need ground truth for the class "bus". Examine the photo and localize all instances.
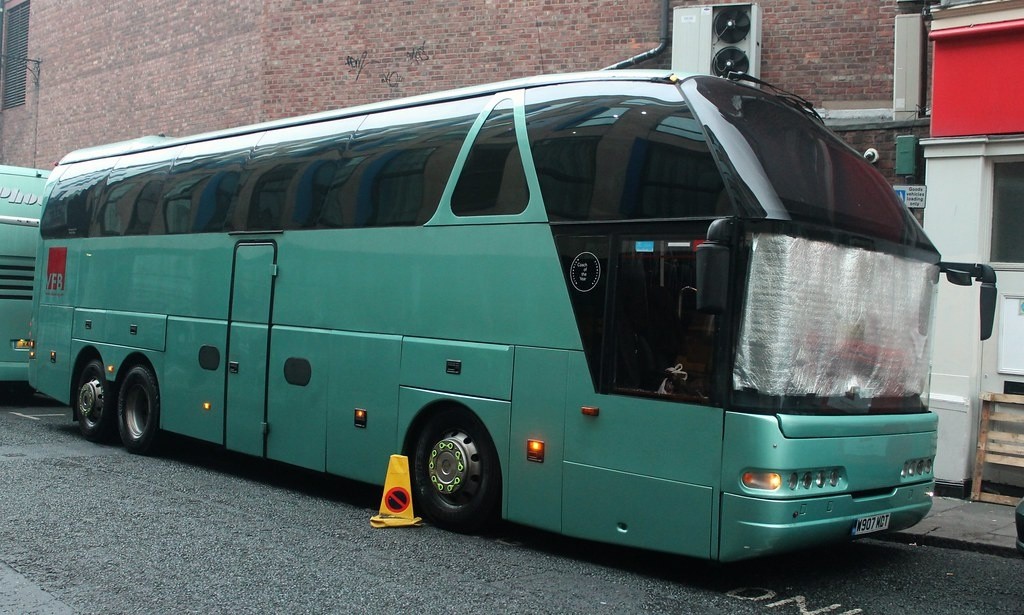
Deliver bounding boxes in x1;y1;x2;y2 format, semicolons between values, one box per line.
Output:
46;68;997;571
0;165;54;382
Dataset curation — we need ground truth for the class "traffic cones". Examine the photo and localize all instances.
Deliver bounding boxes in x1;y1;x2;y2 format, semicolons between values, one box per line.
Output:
370;453;423;530
